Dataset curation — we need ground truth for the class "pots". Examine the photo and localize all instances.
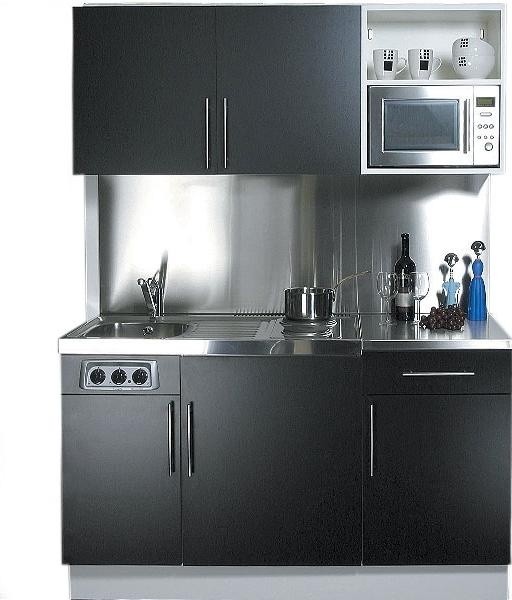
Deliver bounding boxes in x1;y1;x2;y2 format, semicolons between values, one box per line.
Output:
283;267;371;322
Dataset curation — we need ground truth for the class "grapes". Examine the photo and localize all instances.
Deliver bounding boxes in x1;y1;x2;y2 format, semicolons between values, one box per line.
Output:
419;303;467;332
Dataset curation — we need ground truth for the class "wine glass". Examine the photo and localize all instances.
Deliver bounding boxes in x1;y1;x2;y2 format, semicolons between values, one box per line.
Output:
377;272;398;327
407;272;429;326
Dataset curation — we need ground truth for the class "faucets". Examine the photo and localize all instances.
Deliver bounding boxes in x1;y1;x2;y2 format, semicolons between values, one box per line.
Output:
137;277;160;318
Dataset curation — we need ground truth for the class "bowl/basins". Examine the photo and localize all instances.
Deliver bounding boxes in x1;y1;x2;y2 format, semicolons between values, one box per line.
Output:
451;37;496;80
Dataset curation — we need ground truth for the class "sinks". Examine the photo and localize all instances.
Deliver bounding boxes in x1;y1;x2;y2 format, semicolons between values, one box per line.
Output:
66;316;190;339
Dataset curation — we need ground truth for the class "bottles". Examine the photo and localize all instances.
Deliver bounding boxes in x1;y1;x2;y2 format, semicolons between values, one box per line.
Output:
393;232;415;322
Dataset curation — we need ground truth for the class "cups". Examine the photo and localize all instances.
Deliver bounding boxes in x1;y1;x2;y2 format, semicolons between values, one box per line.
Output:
406;46;442;80
372;47;407;80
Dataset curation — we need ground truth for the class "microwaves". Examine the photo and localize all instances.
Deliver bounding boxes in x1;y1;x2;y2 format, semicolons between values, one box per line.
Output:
365;84;501;168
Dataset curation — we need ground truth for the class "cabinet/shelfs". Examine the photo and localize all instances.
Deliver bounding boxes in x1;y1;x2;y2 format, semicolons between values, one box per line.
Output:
61;352;362;567
361;4;505;175
362;351;512;568
73;5;361;176
